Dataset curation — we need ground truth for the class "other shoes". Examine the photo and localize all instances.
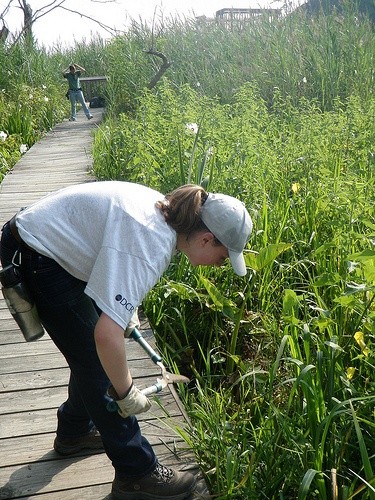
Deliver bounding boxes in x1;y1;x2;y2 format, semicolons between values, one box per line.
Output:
88;116;94;120
69;116;75;121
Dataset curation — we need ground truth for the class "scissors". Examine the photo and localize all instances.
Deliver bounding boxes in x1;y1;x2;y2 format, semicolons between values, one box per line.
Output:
127;325;190;397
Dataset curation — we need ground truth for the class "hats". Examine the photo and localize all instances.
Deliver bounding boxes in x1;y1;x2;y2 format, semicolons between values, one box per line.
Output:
69;64;76;70
195;192;253;277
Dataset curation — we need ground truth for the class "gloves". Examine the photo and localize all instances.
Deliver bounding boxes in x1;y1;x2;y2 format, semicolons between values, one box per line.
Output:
124;307;141;339
107;380;152;418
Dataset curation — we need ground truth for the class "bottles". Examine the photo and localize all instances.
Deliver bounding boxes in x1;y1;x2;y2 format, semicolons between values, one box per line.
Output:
0;265;45;342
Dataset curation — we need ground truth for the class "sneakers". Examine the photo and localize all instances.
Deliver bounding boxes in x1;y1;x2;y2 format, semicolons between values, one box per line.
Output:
110;462;198;500
54;427;105;457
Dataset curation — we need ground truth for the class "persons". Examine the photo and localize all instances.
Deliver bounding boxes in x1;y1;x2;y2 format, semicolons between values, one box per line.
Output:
0;180;255;500
61;63;92;121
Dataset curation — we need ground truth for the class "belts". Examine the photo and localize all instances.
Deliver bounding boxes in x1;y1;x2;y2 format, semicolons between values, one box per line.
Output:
9;217;35;253
69;88;80;91
